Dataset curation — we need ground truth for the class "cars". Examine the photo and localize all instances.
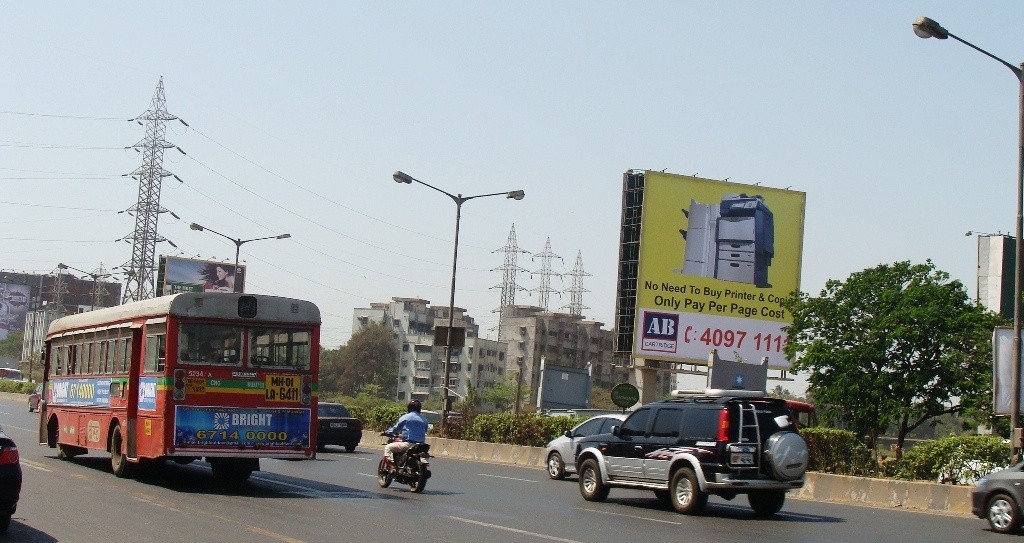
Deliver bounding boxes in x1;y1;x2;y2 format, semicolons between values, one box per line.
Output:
545;413;634;478
969;456;1024;535
313;400;364;454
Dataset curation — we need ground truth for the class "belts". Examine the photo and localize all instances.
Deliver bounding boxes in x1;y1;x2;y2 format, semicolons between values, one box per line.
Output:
407;440;424;444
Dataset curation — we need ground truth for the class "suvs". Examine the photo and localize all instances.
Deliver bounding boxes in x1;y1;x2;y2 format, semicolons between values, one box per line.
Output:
574;387;809;515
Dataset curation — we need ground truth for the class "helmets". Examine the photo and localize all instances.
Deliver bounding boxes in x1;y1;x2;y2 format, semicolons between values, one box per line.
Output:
408;399;421;412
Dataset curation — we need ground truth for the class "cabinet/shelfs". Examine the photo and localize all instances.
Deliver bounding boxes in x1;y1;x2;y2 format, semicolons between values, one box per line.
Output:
715;216;777;288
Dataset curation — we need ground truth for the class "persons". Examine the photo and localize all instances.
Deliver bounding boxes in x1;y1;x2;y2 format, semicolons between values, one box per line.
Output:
212;265;234;290
383;400;429;467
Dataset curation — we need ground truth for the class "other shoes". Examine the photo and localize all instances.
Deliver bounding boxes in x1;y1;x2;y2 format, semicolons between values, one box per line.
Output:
385;464;394;473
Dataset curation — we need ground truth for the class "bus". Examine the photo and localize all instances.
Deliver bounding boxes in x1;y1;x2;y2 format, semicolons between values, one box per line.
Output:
38;291;320;486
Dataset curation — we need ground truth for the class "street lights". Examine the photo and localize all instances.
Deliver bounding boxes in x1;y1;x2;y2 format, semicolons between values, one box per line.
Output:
913;16;1017;466
395;169;525;429
191;222;294;293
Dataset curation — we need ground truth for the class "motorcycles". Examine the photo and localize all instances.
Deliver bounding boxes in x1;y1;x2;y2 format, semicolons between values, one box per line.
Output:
377;429;432;495
